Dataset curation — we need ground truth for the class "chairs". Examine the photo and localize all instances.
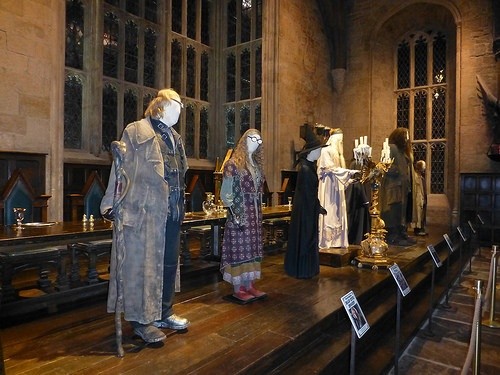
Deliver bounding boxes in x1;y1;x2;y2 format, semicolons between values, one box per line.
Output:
67;169;105;220
186;175;210;212
0;168;49;228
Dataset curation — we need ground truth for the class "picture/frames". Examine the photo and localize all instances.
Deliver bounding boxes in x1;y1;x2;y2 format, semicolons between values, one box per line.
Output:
427;244;442;267
456;226;467;241
389;264;411;297
341;291;370;339
477;214;484;224
468;220;477;233
442;234;456;252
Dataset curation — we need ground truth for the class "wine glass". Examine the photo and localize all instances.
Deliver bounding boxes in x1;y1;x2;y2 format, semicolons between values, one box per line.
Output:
13;208;25;229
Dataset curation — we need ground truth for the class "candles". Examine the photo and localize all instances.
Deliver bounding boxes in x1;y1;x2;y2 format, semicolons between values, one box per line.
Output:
381;138;391;158
355;136;367;147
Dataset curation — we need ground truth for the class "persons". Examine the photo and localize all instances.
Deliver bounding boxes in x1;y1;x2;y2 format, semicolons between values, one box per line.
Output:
317;128;361;249
220;128;269;304
100;88;191;344
281;124;331;278
346;127;428;244
349;307;366;330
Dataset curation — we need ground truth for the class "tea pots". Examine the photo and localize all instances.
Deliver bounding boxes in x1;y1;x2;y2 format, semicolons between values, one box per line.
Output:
203;200;216;215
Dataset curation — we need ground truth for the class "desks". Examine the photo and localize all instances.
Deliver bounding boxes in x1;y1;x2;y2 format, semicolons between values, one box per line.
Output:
0;205;291;330
